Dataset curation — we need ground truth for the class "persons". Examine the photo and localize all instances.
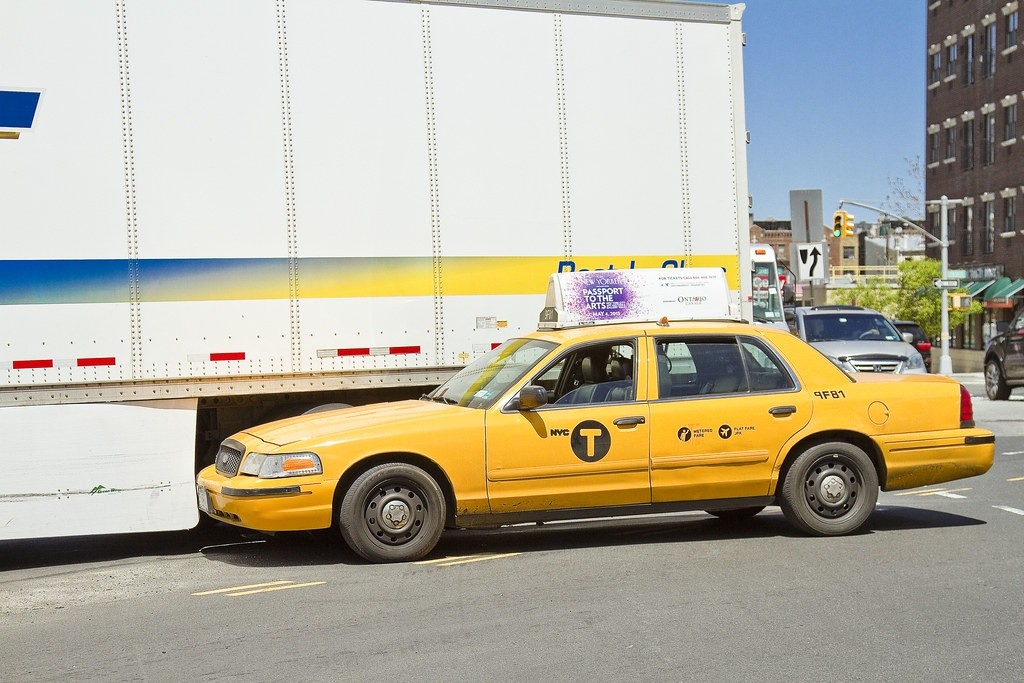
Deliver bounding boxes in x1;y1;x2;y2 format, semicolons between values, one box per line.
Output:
853;318;880;338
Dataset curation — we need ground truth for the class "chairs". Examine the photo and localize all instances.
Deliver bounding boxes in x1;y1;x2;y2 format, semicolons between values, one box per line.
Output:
710;359;740;393
573;357;606;405
605;359;633;402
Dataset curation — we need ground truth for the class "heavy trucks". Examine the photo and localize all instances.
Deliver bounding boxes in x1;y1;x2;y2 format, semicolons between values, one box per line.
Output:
0;0;754;540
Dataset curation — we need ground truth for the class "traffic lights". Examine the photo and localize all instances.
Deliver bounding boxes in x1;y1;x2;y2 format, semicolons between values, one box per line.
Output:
834;212;845;240
845;214;854;237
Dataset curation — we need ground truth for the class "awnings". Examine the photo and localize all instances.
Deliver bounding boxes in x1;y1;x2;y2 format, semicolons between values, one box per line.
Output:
960;280;995;297
992;279;1024;303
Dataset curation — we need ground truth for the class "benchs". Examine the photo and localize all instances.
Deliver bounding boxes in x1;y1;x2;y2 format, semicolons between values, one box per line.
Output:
737;371;788;392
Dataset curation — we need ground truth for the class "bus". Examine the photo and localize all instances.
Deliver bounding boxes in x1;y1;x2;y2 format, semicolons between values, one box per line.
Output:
749;243;797;336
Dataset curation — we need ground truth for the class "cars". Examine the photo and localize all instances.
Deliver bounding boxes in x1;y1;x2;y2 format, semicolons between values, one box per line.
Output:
196;265;996;537
783;306;927;377
980;303;1024;399
876;323;932;375
